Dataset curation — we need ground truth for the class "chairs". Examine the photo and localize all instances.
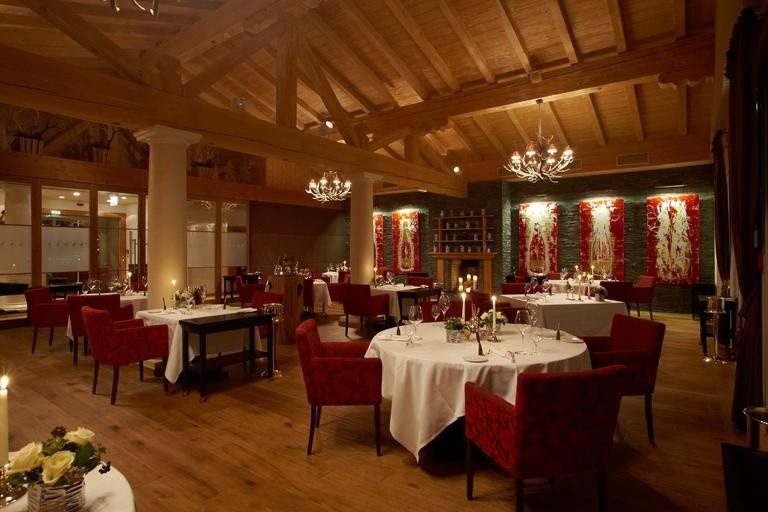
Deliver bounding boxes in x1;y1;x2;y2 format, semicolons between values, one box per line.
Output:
320;276;330;283
304;278;314;306
338;270;350;282
500;283;542;294
600;281;632;316
236;277;265;308
408;277;434;289
251;291;284;336
418;300;471;322
407;272;428;278
323;283;343;313
513;275;542;284
630;275;655;320
382;267;393;281
464;362;625;512
132;282;147;291
296;320;383;456
546;273;568;280
576;314;666;442
470;291;528;324
66;294;134;367
80;306;171;405
24;287;69;353
340;283;389;337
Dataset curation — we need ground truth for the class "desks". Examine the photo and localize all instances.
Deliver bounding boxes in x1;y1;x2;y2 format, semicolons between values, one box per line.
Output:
223;275;260;303
181;311;275;402
227;266;248;288
268;273;304;342
698;310;729;355
690;284;715;319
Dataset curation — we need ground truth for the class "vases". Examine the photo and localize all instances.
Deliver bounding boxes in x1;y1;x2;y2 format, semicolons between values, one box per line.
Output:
27;479;87;511
114;290;125;295
486;323;500;334
579;279;587;283
177;302;186;308
594;293;605;302
446;328;463;343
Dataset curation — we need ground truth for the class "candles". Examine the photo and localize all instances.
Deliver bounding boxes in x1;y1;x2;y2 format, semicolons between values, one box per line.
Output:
1;372;10;469
466;274;470;293
127;272;131;290
459;278;463;292
343;261;346;270
577;275;581;300
592;266;595;276
462;293;465;323
374;267;377;283
587;278;590;299
172;280;176;308
492;295;497;333
575;265;578;272
472;275;478;290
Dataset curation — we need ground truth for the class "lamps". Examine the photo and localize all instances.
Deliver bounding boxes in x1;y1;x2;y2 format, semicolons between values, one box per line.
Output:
504;98;575;184
110;196;118;206
304;120;352;203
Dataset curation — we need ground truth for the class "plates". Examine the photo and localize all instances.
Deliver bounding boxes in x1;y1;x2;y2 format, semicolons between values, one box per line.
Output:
463;355;488;362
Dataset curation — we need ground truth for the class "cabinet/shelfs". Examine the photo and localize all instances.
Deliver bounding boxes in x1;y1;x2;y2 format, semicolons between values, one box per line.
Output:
429;213;496;292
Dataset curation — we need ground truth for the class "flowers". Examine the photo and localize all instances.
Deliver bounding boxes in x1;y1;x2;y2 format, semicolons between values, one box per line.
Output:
108;279;126;288
588;283;605;292
175;290;191;301
2;424;111;505
377;275;383;283
477;310;507;325
579;273;589;281
441;317;467;330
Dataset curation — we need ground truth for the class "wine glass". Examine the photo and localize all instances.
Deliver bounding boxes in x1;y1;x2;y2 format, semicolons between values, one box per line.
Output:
432;305;440;326
406;324;415;348
525;306;539;334
142;276;147;296
438;294;450;325
531;278;538;296
530;327;543;353
515;310;531;346
542;279;550;303
524;283;531;298
409;305;423;338
